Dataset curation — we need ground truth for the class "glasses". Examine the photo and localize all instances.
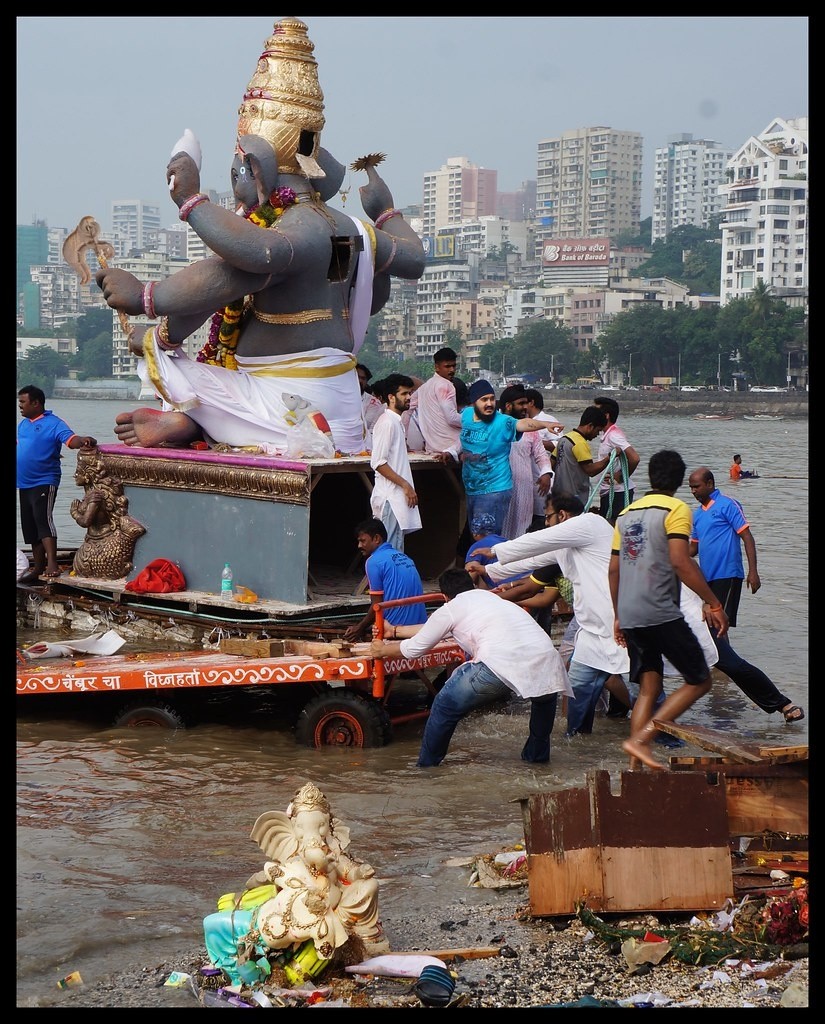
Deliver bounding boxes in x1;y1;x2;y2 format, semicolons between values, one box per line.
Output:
545;509;566;522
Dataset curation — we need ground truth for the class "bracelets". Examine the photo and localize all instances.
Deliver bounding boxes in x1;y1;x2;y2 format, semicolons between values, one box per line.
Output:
490;546;496;556
711;603;722;611
374;208;402;230
142;282;158;321
510;581;513;588
394;625;398;640
156;323;180;351
179;191;208;222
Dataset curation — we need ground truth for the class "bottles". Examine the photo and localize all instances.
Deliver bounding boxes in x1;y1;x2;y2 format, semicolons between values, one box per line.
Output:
221;564;233;598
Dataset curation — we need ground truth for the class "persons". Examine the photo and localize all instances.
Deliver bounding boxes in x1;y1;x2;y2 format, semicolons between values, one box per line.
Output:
678;468;804;724
729;454;752;479
388;348;565;539
200;782;386;980
608;449;729;773
342;518;427;643
595;395;641;525
17;384;99;580
69;450;127;578
369;567;575;771
96;17;425;451
372;374;423;552
353;362;386;433
548;405;622;511
465;489;666;737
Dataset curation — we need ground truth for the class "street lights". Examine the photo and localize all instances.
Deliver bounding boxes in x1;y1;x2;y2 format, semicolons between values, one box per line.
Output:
788;350;799;387
543;352;562;382
629;352;641;385
718;353;728;387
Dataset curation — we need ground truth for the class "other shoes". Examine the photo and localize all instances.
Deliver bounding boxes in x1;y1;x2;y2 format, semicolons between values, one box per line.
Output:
607;693;629;719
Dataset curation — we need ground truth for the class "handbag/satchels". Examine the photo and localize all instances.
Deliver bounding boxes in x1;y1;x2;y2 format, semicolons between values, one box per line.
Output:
126;558;186;594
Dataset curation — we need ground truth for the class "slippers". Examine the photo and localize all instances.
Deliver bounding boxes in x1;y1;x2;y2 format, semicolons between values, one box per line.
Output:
415;965;455;1006
784;705;804;722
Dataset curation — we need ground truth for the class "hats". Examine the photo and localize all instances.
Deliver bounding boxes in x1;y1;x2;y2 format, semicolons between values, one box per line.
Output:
500;384;526;409
471;513;496;533
469;380;494;403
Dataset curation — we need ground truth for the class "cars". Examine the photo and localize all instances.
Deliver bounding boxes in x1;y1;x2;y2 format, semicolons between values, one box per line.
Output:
498;383;797;392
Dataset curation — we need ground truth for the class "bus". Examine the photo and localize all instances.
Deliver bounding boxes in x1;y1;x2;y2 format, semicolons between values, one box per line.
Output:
577;378;602;389
